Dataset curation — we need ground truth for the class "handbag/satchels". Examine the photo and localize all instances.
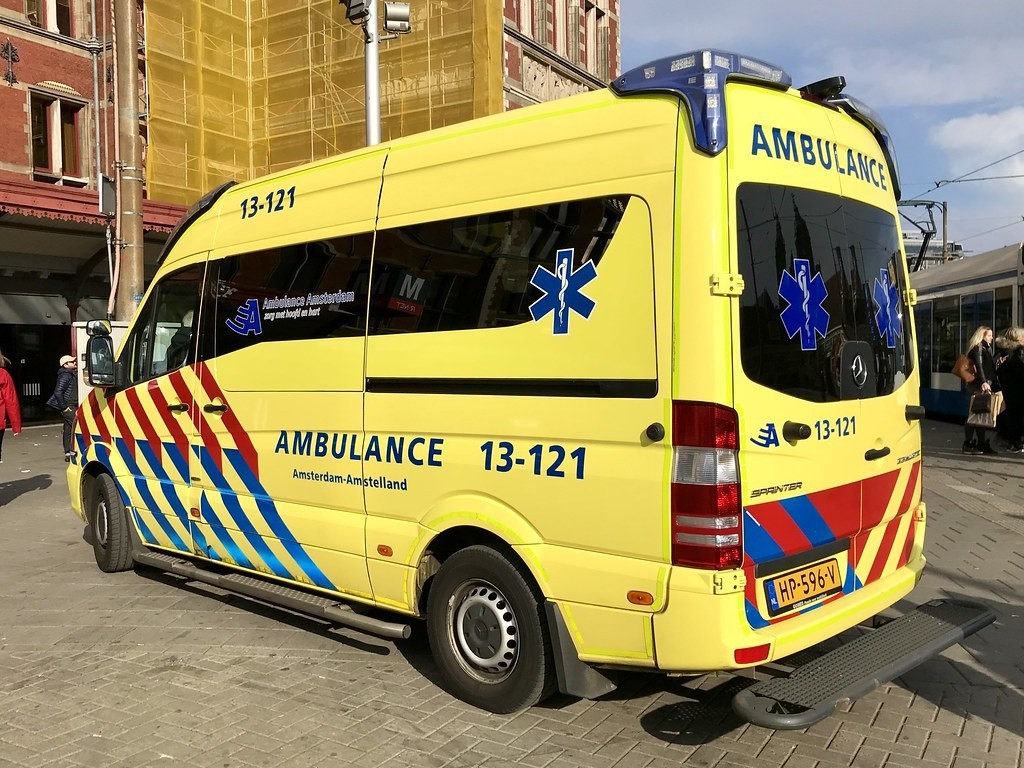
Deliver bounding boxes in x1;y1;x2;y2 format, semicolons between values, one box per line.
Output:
952;345;980;383
966;390;1006;429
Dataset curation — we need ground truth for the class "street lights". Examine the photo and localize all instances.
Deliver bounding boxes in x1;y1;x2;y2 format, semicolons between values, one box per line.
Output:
339;0;413;148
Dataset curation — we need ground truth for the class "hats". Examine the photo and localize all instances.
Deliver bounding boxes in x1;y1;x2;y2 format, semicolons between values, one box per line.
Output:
60;355;76;366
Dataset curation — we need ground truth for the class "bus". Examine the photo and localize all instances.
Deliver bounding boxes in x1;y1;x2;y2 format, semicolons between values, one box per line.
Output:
908;242;1024;421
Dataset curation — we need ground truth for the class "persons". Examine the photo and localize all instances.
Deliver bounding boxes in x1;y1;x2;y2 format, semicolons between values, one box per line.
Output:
45;355;78;462
960;325;1003;457
983;324;1024;455
0;351;20;464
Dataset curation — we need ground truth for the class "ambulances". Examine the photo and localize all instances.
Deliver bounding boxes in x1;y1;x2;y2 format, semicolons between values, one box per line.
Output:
67;48;997;732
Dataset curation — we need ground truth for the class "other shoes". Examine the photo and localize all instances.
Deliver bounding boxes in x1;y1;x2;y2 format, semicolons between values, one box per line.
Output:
1005;445;1024;453
962;439;998;455
